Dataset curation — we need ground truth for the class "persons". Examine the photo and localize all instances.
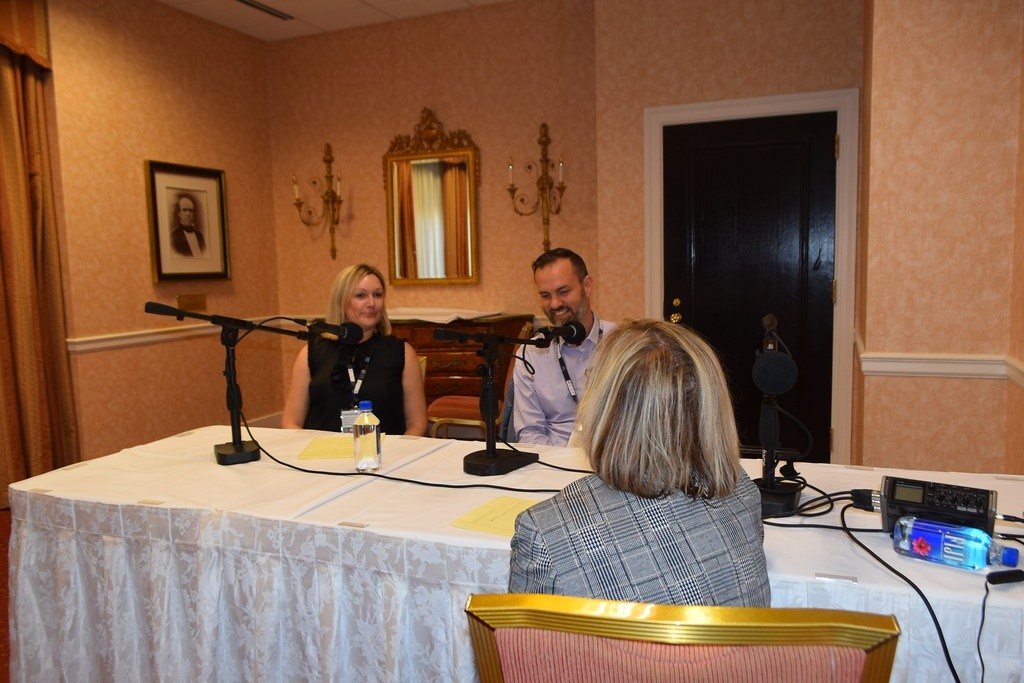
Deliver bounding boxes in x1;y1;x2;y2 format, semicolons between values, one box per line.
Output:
169;194;207;257
281;263;429;437
507;319;773;608
513;247;618;449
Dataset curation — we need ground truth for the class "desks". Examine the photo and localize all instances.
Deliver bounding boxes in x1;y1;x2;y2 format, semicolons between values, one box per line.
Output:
4;424;1024;683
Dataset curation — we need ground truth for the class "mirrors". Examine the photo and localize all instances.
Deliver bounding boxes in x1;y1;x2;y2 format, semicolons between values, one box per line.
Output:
383;107;482;286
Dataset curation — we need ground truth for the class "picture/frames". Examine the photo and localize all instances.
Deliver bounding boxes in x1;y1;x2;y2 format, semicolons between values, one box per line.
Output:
145;160;231;283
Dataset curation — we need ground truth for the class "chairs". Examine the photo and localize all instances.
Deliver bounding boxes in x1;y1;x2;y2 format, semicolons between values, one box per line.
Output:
427;322;533;442
464;592;902;683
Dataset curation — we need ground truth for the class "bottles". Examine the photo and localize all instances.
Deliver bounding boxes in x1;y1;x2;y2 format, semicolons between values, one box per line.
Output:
353;401;382;473
894;516;1019;572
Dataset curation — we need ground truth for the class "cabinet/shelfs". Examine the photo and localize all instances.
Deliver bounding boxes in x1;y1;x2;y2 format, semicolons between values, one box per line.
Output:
388;313;536;405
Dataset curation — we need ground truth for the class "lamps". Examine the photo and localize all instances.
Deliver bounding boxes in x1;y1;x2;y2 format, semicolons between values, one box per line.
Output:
507;123;566;253
293;143;344;260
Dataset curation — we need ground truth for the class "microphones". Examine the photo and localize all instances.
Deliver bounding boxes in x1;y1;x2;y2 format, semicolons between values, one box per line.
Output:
753;350;798;396
537;320;586;344
294;318;363;346
